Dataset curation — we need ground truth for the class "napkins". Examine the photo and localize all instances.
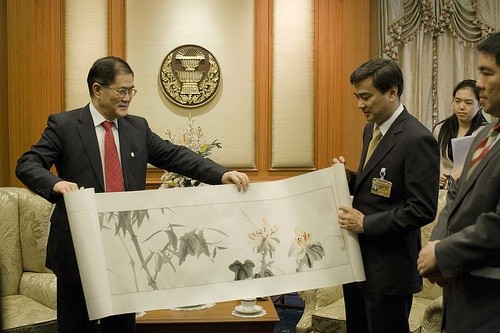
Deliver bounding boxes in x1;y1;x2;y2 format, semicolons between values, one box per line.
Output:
136;312;146;318
231;306;268;318
166;302;217;311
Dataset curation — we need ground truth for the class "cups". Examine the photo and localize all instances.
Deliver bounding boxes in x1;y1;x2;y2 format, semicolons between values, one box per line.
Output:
241;297;256;310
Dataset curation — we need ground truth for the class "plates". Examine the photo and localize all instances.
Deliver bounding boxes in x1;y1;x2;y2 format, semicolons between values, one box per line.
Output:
235;305;262;314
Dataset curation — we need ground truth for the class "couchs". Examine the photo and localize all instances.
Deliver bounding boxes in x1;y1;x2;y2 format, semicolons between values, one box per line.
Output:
295;189;448;333
0;187;57;333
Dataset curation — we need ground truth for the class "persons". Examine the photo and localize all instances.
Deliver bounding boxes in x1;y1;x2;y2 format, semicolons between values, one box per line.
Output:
15;57;249;332
332;57;440;333
432;79;487;189
417;32;500;333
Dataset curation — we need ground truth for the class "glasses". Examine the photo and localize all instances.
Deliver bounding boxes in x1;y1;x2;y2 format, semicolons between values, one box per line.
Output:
96;81;138;98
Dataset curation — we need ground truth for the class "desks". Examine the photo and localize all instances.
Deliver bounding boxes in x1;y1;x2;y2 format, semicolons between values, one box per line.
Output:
135;296;280;333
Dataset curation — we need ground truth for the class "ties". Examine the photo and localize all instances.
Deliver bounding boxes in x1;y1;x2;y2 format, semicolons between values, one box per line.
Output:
466;120;500;186
100;121;126;193
363;127;382;170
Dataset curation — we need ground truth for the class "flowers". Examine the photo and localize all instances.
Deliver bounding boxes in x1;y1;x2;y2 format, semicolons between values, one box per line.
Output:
157;113;222;190
